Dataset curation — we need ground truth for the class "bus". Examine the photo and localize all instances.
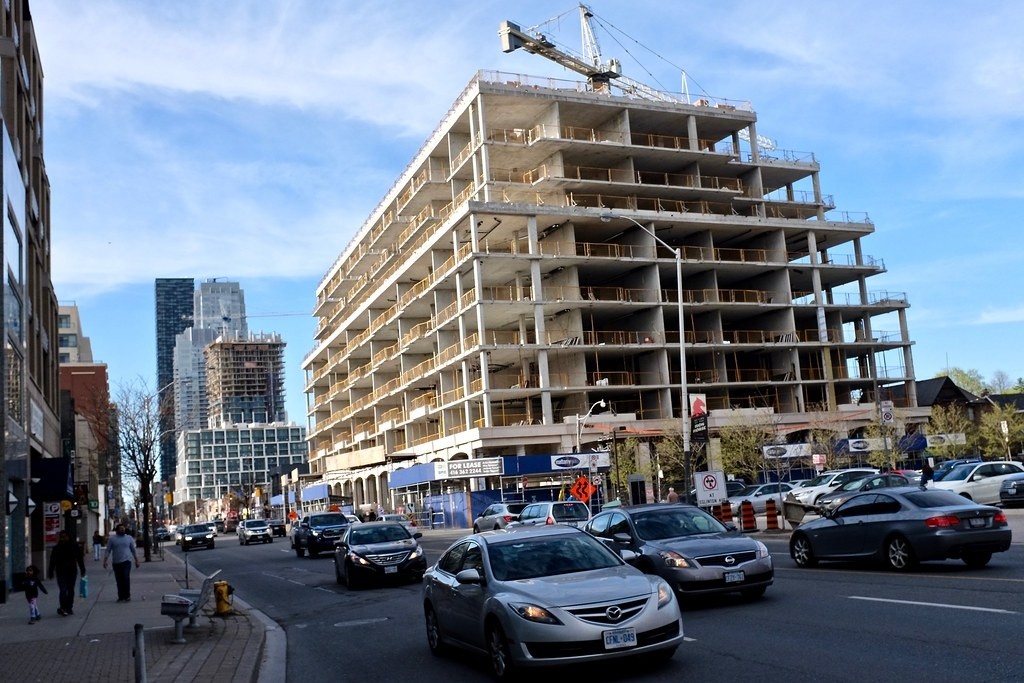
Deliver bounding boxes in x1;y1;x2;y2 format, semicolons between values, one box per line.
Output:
511;500;592;529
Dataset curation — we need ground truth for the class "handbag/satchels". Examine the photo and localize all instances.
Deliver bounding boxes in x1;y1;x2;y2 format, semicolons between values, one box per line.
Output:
80;575;88;599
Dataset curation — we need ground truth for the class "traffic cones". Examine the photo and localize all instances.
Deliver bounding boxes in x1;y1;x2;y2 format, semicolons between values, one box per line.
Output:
740;499;760;533
722;499;732;522
763;498;782;533
713;506;722;522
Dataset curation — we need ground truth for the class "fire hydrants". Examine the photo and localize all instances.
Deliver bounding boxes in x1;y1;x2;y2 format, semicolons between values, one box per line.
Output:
213;580;235;615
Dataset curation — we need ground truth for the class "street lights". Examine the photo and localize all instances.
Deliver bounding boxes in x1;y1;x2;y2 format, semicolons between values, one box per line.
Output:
576;398;606;454
144;376;180;424
600;211;692;503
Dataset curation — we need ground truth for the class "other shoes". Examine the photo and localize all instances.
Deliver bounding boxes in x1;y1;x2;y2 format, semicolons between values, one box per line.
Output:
116;597;131;602
35;614;41;621
29;617;36;624
56;608;73;616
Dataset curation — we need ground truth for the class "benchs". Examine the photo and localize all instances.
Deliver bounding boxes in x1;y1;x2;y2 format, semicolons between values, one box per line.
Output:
161;568;223;644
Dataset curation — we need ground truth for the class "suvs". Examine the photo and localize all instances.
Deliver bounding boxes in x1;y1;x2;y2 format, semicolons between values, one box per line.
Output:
472;500;531;534
289;512;363;560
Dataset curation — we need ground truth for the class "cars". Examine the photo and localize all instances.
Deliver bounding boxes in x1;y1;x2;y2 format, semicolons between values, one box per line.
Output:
580;503;775;604
788;485;1013;572
421;524;684;681
678;458;1024;516
333;520;428;591
155;518;287;552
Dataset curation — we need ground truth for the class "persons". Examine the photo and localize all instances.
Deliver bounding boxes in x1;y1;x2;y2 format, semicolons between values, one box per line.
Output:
920;463;934;487
488;548;519;581
93;530;102;561
47;529;87;617
103;523;141;602
427;507;435;529
24;565;49;625
358;514;365;523
287;507;299;533
666;487;680;503
379;510;384;515
368;509;375;522
